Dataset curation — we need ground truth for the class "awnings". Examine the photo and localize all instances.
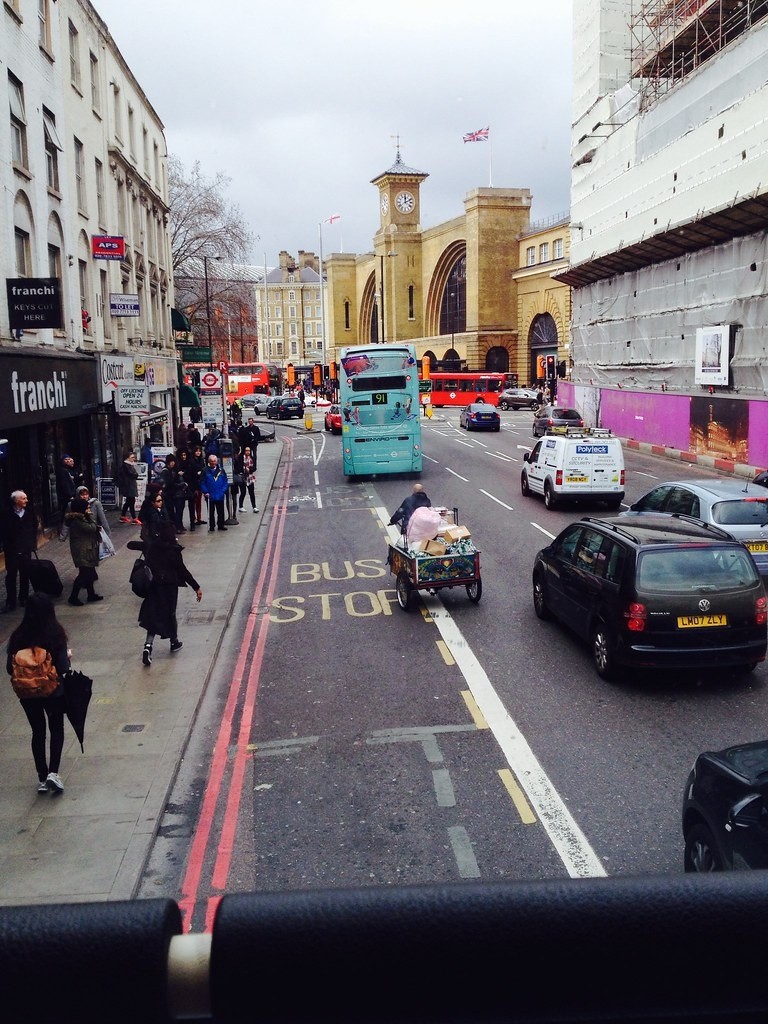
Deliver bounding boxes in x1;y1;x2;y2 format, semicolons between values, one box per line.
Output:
171;308;191;333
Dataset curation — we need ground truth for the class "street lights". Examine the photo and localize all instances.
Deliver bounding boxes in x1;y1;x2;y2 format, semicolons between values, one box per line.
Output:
263;251;271;364
317;215;341;365
366;250;399;344
203;253;225;372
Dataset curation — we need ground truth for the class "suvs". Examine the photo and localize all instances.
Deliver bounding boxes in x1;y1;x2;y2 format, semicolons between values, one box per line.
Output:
681;740;768;872
498;388;548;411
533;513;768;681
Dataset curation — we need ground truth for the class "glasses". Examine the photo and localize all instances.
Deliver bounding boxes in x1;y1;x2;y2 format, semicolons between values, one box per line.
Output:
154;499;163;502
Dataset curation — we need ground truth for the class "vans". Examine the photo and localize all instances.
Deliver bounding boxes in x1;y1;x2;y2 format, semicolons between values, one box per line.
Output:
520;428;625;511
618;472;768;575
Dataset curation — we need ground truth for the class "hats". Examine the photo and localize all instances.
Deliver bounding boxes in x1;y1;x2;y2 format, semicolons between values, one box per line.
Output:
146;483;163;495
71;498;89;513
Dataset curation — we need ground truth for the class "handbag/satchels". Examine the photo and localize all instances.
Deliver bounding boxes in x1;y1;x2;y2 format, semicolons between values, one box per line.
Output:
173;480;194;499
112;465;128;488
233;471;245;484
95;526;117;567
129;557;154;599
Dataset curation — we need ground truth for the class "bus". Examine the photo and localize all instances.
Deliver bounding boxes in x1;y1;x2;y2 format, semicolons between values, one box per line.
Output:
428;373;519;408
339;344;423;481
183;363;269;409
419;380;444;408
267;365;283;396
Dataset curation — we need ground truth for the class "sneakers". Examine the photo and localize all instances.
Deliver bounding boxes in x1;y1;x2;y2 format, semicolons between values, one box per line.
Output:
170;642;184;652
239;508;247;512
68;596;84;606
118;517;131;522
180;527;188;531
131;517;143;524
87;595;103;601
0;603;16;613
209;526;214;531
253;508;260;513
218;527;227;530
190;523;196;531
18;599;27;607
174;530;185;534
196;521;206;525
46;772;65;793
142;644;152;665
37;780;48;793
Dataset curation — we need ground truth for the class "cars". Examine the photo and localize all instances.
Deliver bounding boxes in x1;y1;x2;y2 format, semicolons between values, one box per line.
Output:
324;406;343;435
266;398;304;419
240;394;268;408
460;404;501;433
254;397;282;415
532;408;584;437
281;392;316;408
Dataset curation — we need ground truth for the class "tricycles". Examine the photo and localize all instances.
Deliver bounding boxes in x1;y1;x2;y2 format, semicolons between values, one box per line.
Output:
387;522;482;610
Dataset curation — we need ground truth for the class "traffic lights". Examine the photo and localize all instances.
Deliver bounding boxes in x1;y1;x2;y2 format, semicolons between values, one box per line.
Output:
323;365;330;380
546;355;557;380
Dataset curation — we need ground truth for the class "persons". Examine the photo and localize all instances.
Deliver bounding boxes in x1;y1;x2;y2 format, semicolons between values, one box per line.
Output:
53;377;340;542
5;593;73;794
64;498;104;606
432;375;559;408
388;483;431;535
137;518;203;666
0;490;39;611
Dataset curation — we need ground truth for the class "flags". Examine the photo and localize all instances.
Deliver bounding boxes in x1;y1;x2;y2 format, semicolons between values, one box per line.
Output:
322;215;341;224
463;126;489;142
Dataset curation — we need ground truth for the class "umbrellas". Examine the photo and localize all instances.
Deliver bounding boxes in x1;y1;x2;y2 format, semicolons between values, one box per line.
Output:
63;655;94;754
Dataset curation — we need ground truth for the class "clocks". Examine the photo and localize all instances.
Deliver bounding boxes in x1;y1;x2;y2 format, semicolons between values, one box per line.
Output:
379;192;390;216
394;190;416;214
